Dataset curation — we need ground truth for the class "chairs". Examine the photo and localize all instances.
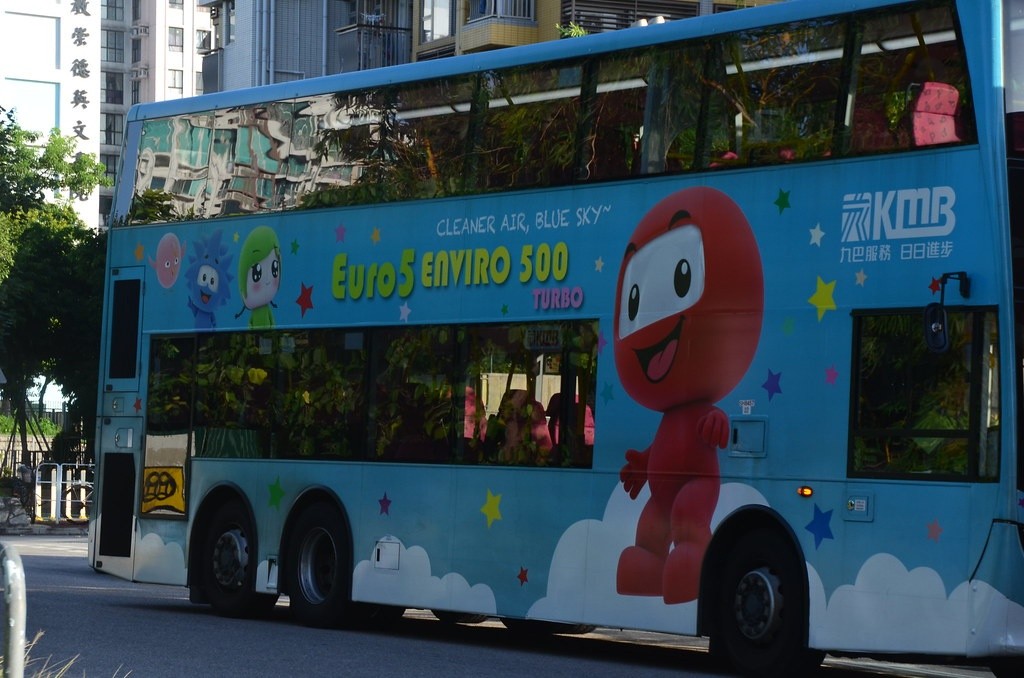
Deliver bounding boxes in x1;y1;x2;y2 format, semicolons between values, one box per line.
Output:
339;370;594;468
579;83;959;181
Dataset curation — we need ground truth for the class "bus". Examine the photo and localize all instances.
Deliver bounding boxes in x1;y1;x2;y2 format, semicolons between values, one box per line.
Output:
87;0;1024;678
